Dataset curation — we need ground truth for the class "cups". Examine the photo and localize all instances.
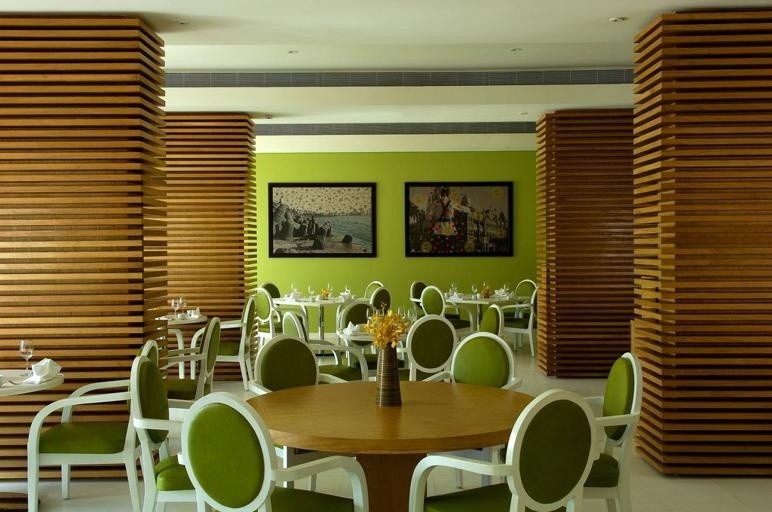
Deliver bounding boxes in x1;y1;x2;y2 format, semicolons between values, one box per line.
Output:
366;304;417;323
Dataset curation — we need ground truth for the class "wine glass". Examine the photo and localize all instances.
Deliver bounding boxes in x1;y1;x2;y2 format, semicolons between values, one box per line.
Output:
289;282;354;304
171;296;199;320
20;339;34;377
447;280;511;300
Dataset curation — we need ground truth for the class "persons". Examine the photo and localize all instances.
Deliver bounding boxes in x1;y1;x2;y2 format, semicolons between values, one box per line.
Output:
427;192;433;210
432;189;440;203
498;209;506;228
452;196;477;253
491;208;498;226
426;186;471;253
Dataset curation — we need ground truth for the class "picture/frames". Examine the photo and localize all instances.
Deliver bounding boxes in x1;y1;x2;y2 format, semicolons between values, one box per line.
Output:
403;181;514;258
267;182;377;259
